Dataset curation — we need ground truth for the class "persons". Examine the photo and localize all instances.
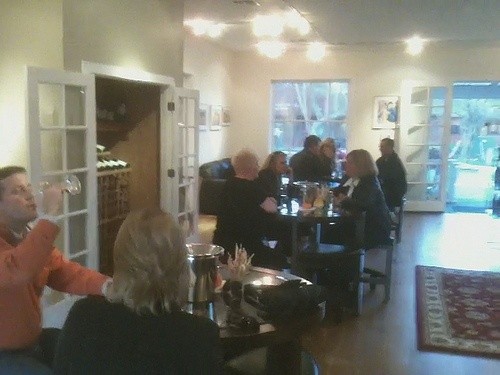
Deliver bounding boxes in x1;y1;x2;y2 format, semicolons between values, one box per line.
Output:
375;138;407;211
54;206;220;375
1;166;114;375
319;137;336;176
257;150;293;269
289;135;323;180
324;149;391;253
213;149;282;271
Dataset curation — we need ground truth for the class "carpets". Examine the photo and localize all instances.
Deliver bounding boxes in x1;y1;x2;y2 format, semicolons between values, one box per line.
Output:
415;264;499;359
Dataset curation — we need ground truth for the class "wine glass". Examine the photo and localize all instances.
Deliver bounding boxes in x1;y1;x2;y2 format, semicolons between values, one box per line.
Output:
25;173;82;206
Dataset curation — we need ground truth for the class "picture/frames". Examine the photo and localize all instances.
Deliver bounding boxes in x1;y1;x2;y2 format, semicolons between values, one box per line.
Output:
210;106;222;129
371;94;401;129
199;103;210;129
222;106;232;124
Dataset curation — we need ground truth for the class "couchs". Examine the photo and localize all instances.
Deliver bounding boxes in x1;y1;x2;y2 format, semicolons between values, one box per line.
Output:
199;157;233;214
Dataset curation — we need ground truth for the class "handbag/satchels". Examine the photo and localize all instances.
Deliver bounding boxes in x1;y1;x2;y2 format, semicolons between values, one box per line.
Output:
244;278;327;325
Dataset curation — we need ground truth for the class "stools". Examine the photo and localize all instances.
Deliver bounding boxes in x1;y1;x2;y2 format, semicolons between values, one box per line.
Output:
343;243;394;316
387;200;404;242
300;242;345;285
